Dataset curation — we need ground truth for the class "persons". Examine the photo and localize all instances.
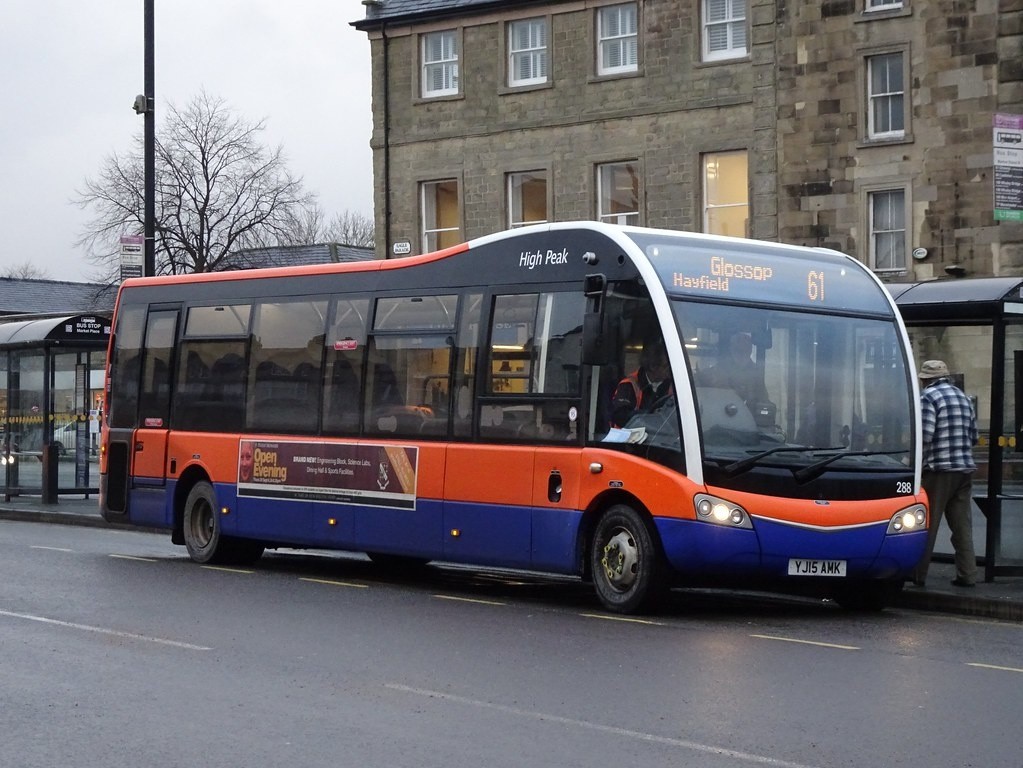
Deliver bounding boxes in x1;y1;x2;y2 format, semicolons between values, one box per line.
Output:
797;402;868;450
916;360;979;589
698;332;775;435
607;342;674;430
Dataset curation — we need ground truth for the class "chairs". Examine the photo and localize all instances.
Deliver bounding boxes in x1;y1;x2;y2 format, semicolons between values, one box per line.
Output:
121;350;449;437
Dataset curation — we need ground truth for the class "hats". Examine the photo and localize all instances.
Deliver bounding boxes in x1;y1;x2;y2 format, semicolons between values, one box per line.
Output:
643;343;668;368
917;360;950;379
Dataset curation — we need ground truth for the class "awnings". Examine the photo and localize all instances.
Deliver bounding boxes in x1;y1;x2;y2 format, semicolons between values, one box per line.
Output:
368;295;540;350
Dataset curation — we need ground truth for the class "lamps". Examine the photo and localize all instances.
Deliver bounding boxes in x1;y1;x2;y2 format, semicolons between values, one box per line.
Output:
944;265;966;278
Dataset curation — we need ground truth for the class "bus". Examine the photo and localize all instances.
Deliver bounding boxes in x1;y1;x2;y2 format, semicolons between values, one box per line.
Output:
97;220;931;616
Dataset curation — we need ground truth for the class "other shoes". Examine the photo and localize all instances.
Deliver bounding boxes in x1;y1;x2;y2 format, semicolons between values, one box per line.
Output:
904;576;925;586
950;577;975;587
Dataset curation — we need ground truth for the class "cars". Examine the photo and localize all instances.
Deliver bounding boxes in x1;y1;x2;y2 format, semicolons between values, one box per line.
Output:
36;413;102;462
